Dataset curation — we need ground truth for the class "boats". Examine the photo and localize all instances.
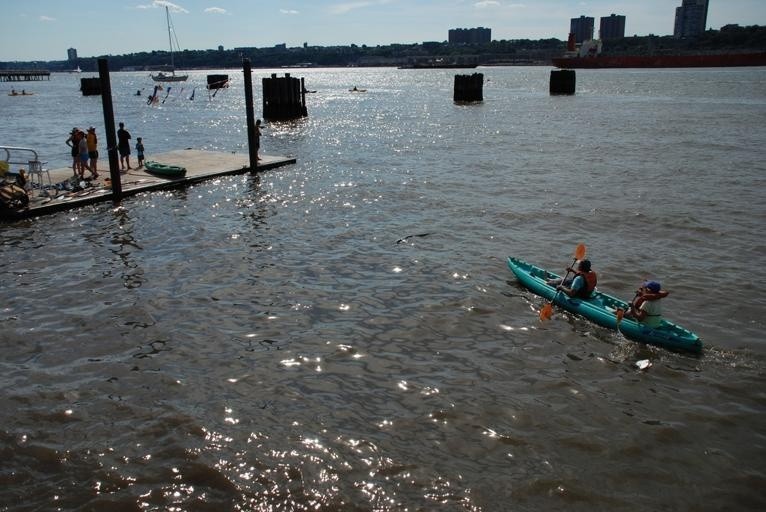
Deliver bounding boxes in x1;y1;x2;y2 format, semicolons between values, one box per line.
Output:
7;93;34;96
507;255;704;352
144;158;187;177
349;90;367;92
151;5;188;81
300;91;317;93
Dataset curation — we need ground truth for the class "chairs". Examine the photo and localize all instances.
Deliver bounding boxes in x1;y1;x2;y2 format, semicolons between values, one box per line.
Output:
25;160;52;189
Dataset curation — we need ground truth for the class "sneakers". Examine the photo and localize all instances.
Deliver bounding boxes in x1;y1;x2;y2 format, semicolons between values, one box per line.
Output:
73;171;99;181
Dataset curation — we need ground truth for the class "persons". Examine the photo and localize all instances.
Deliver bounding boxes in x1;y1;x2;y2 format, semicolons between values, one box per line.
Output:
86;125;98;176
544;259;597;299
11;90;17;95
605;281;662;329
19;168;28;181
117;122;132;170
254;119;263;161
22;90;26;94
65;126;83;178
76;129;99;180
135;137;145;167
353;86;357;90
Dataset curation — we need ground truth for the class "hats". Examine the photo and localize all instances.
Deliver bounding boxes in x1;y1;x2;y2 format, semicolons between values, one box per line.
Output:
643;280;661;292
70;125;97;136
574;258;592;268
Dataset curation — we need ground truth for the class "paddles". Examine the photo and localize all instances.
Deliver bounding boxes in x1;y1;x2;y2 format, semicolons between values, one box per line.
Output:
40;169;147;205
539;244;585;320
617;280;648;320
